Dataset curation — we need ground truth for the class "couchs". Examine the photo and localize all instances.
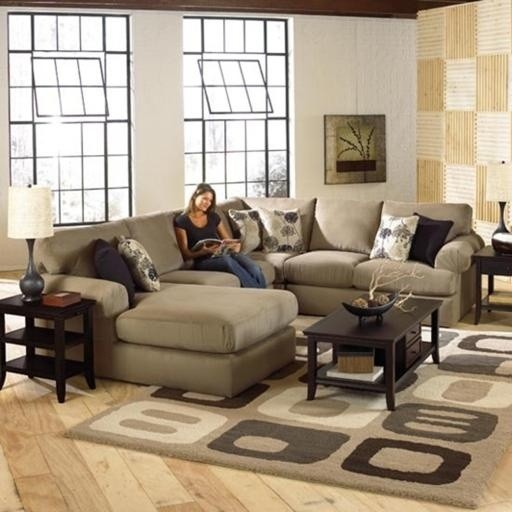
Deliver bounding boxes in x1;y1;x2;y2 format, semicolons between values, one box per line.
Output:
34;198;485;397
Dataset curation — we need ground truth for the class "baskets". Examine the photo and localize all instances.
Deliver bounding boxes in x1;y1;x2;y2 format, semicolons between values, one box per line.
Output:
336;346;375;373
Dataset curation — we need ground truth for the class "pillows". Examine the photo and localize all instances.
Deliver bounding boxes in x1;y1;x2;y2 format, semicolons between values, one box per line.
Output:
227;209;263;255
94;240;136;306
370;215;419;262
119;238;160;292
258;208;305;253
409;212;454;267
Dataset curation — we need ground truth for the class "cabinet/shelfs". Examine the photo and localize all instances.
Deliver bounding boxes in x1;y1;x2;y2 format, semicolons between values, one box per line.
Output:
472;244;512;324
0;292;97;403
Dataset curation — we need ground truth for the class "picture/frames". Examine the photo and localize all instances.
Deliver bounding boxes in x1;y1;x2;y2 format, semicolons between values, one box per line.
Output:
324;114;385;185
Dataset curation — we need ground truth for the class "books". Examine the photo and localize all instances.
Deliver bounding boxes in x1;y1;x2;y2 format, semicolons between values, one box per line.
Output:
326;364;383;382
190;240;240;258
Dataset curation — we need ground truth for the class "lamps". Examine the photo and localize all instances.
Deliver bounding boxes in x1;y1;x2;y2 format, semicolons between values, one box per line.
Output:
486;163;512;253
9;186;54;302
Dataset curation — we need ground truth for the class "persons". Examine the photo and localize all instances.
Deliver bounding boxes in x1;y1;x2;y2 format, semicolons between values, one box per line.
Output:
173;184;266;287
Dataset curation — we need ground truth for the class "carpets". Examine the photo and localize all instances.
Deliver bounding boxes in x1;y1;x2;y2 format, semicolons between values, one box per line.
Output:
64;319;511;508
